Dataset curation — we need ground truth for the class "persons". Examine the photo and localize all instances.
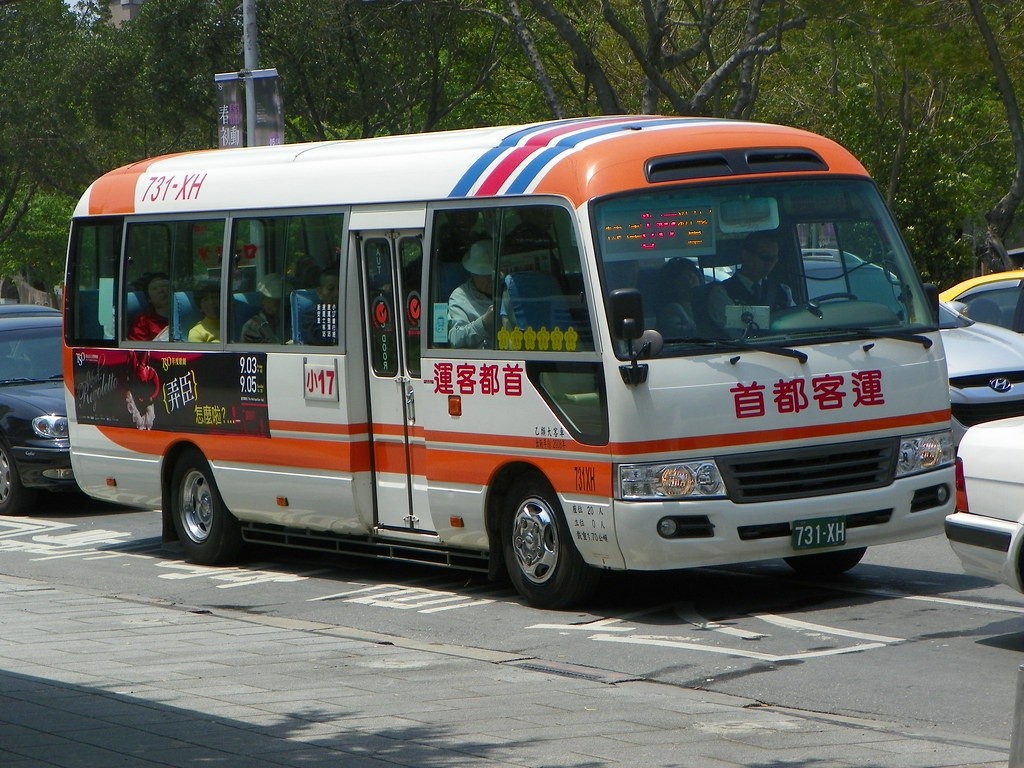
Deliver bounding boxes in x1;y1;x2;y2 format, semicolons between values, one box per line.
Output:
661;257;703;291
238;274;302;344
447;239;516;349
970;298;1003;326
504;204;563;276
292;252;326;289
707;230;797;338
187;279;234;344
128;270;175;342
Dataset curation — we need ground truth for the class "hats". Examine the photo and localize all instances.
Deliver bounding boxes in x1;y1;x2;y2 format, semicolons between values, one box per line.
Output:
255;273;294;298
462;239;493;275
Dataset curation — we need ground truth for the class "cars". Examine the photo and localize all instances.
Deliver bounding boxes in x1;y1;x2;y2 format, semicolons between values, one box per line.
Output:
938;270;1024;335
945;417;1024;598
692;250;1024;447
0;304;76;515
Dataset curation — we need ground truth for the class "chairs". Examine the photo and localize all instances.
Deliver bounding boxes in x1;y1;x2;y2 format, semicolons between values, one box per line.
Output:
693;281;726;336
632;265;671;336
78;260;588;351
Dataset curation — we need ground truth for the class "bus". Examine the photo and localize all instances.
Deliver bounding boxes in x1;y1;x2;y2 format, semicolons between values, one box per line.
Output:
60;116;958;609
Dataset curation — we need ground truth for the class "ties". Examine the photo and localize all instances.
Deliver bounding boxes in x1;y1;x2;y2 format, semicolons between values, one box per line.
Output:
751;283;761;307
